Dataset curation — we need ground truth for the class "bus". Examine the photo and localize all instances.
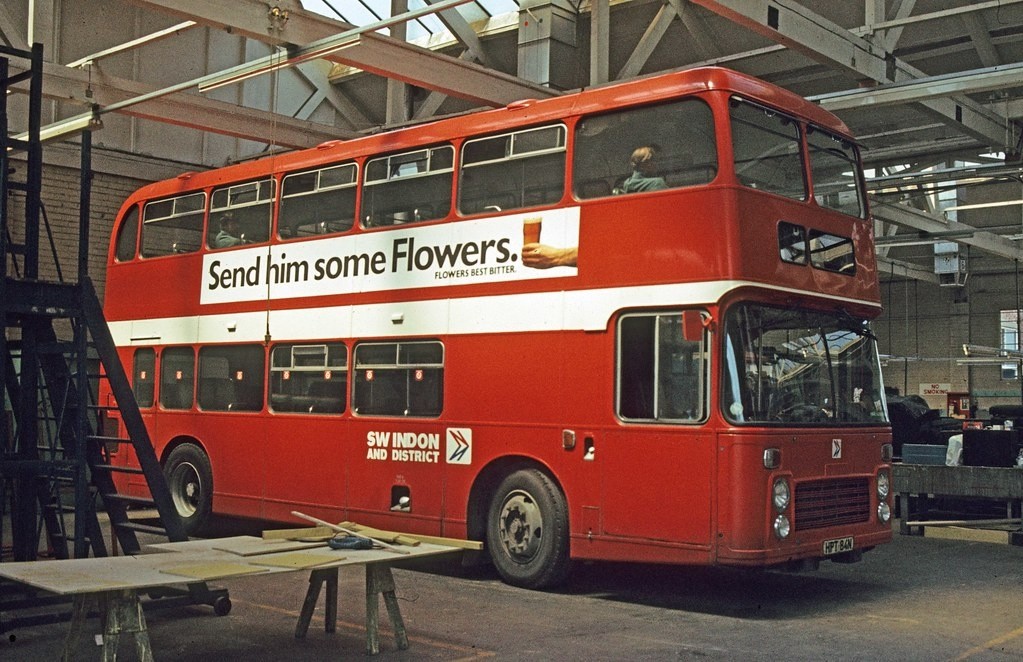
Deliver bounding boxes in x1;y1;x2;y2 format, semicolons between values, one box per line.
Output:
96;62;899;584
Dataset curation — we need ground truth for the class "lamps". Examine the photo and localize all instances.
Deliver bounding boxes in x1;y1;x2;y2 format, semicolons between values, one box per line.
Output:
89;104;105;129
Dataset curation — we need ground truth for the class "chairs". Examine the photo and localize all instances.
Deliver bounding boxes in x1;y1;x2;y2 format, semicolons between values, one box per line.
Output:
116;175;625;263
155;403;416;416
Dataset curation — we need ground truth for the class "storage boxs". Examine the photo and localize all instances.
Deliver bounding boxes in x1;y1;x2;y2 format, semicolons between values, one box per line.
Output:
901;429;1019;464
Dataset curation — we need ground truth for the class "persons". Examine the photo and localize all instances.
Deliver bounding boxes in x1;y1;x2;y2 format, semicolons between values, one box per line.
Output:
521;241;578;269
215;212;258;247
620;147;670;194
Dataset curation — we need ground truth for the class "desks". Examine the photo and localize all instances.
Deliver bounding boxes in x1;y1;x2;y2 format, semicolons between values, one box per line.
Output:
1;534;462;662
892;461;1023;543
